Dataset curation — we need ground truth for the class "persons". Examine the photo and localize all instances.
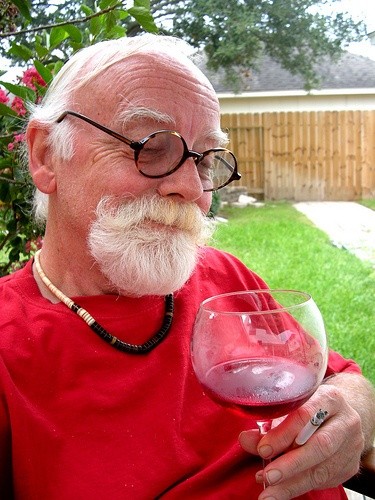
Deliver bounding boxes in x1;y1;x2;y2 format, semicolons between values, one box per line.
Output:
0;30;375;500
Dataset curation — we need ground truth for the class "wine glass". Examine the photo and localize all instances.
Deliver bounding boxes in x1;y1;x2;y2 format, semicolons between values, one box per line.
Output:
190;288;330;491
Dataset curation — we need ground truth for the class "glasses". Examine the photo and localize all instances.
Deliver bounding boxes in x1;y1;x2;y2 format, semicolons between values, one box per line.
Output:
57;110;243;194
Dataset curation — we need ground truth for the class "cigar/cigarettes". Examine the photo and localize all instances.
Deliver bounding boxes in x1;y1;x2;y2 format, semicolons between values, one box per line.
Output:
286;407;329;454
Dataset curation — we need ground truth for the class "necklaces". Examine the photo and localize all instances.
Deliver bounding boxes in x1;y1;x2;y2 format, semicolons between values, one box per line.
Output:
34;245;174;354
